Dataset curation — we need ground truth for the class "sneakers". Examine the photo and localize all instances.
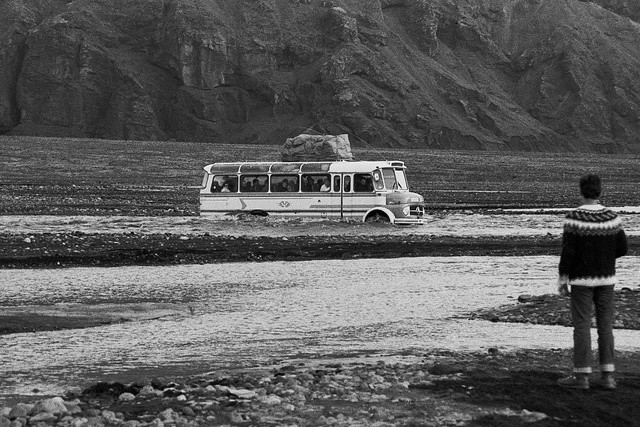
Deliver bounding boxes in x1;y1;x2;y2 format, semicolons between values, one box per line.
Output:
594;375;616;389
556;375;589;389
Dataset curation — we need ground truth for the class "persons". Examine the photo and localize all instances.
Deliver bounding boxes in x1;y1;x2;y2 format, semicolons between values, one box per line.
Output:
557;174;627;391
211;174;370;193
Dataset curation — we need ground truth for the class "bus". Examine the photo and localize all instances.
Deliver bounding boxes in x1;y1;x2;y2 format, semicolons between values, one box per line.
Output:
200;161;425;226
